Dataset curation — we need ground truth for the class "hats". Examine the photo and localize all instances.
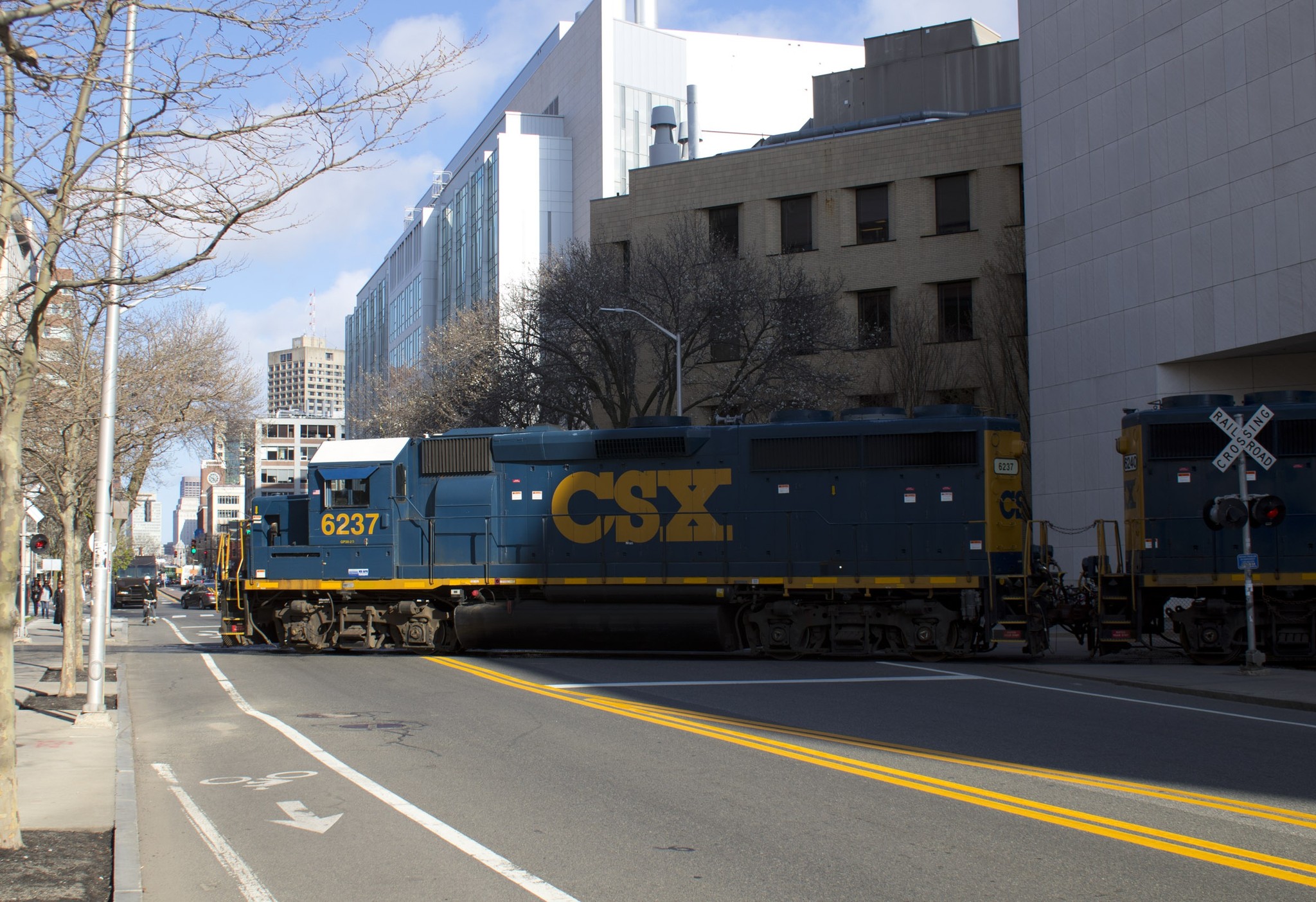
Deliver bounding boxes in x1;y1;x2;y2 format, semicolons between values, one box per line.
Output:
144;576;151;580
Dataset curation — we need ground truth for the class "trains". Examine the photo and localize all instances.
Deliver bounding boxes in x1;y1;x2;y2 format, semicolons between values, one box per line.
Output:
207;390;1316;670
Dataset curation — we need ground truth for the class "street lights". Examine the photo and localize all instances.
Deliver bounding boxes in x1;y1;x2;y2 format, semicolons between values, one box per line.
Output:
82;285;205;715
198;533;219;579
601;306;683;417
217;520;240;572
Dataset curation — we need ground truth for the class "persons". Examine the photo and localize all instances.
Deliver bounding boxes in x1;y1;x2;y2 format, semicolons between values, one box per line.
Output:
141;575;158;623
38;580;53;619
31;579;42;616
53;581;65;632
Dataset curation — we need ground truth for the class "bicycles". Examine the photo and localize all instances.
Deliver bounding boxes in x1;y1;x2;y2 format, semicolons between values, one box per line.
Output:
143;599;156;626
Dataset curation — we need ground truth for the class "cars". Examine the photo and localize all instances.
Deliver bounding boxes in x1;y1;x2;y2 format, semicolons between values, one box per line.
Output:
181;579;221;609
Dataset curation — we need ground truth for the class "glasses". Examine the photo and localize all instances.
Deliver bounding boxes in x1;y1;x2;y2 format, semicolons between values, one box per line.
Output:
58;583;62;585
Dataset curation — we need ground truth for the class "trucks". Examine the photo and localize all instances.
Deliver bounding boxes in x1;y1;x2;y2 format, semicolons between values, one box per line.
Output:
112;556;158;609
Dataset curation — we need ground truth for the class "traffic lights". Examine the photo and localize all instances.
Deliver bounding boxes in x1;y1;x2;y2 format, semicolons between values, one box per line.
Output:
1252;494;1286;527
1218;498;1249;532
245;529;251;536
30;534;50;554
174;551;177;558
204;551;208;559
191;540;197;555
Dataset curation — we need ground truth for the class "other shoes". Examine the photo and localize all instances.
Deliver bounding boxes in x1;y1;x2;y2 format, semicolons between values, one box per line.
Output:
143;619;146;623
61;627;63;632
110;634;114;637
152;620;156;623
